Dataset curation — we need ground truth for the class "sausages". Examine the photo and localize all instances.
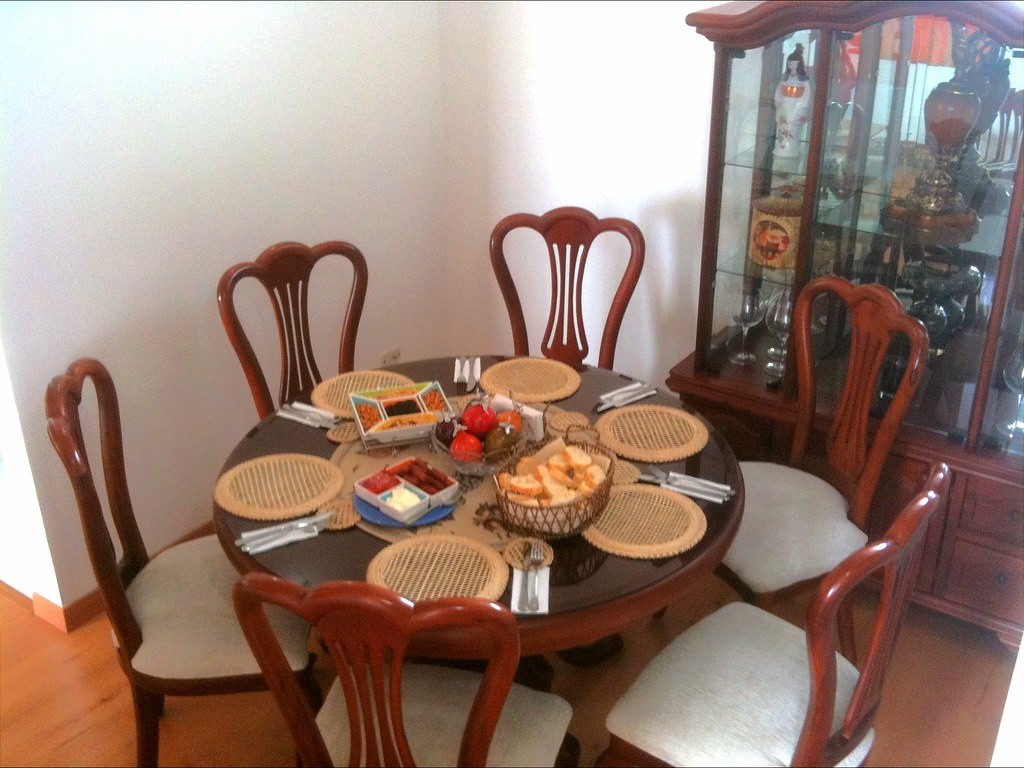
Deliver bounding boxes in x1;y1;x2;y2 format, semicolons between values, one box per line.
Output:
397;459;455;494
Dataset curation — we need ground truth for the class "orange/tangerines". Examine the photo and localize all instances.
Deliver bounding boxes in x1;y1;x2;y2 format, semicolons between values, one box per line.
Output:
494;409;522;431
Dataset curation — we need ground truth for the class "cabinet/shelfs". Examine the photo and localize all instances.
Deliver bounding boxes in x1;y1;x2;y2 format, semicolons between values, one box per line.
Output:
665;1;1024;654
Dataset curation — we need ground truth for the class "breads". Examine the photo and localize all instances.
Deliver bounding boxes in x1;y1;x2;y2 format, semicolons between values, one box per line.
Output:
498;444;606;507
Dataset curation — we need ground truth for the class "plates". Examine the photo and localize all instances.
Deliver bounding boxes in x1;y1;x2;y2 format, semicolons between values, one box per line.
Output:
353;493;455;528
349;379;456;443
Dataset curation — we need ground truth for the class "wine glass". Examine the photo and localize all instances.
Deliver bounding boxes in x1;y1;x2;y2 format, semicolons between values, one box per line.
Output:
765;292;793;377
997;340;1024;440
727;289;766;366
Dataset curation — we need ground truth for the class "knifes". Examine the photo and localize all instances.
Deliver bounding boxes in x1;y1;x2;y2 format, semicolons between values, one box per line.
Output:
234;509;336;547
598;386;658;412
518;541;531;611
631;473;730;502
467;353;476;393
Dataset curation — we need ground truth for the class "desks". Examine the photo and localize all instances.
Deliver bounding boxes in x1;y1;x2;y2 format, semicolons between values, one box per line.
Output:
213;356;745;663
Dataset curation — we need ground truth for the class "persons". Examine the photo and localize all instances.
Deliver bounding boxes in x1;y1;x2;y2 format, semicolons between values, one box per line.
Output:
768;46;813;157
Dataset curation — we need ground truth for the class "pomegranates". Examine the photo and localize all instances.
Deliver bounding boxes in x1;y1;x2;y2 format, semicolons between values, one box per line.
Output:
450;404;495;463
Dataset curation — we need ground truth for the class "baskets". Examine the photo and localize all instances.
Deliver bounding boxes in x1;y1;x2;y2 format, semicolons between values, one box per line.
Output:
492;425;616;540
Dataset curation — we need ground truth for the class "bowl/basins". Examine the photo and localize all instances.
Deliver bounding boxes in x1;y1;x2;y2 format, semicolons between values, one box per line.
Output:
354;455;459;523
429;412;531;476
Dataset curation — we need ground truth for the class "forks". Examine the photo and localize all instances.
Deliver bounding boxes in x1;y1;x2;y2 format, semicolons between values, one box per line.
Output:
241;517;329;553
404;488;463;525
647;461;734;495
527;537;545;611
456;353;467;396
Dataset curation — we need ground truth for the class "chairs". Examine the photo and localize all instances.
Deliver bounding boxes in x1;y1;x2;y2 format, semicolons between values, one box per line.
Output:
488;208;646;372
46;358;323;768
594;462;950;768
231;573;582;768
217;240;369;421
712;274;929;665
